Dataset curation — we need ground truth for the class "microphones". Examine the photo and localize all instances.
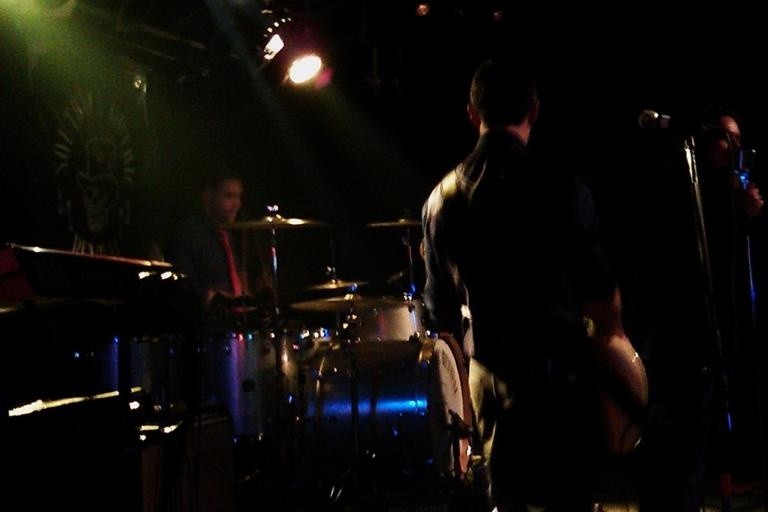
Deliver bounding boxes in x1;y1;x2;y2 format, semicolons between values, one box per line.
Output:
637;109;714;136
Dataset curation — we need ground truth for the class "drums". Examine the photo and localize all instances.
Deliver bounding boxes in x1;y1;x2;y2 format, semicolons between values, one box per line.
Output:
336;298;425;341
302;333;473;486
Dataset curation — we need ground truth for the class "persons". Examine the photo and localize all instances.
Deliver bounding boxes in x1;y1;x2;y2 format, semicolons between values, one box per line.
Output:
420;64;766;511
181;168;278;327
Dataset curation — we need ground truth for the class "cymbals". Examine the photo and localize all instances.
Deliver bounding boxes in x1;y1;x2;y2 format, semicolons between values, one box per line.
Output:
235;215;333;229
367;219;423;226
291;293;393;311
306;278;370;289
